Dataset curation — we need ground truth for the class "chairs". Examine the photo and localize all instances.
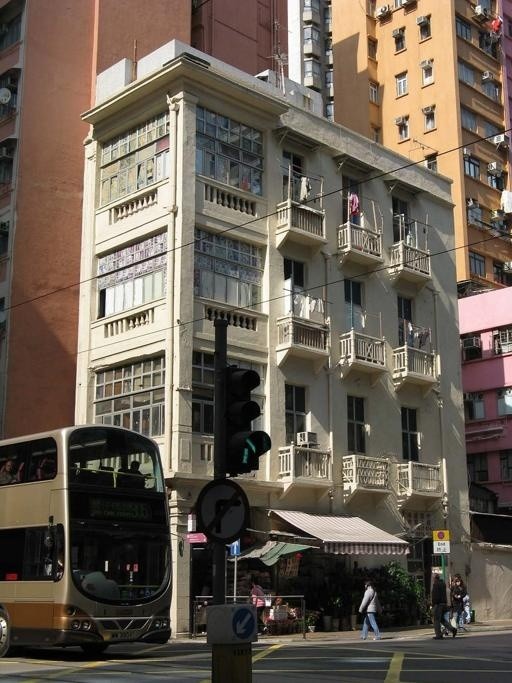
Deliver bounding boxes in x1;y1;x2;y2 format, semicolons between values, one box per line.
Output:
17;459;129;488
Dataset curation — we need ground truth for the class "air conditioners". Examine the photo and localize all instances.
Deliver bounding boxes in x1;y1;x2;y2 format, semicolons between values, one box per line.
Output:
297;432;317;445
462;336;481;351
376;0;512;273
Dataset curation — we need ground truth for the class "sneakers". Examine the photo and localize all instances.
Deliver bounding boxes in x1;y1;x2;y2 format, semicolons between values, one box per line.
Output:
432;626;463;640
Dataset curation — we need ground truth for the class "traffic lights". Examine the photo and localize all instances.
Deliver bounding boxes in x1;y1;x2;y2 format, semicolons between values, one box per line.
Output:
224;367;271;473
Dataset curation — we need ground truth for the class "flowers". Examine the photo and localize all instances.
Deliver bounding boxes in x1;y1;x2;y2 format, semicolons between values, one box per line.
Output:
263;592;320;635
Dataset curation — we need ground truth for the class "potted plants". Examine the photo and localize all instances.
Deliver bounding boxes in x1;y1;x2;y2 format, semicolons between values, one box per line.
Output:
320;557;435;632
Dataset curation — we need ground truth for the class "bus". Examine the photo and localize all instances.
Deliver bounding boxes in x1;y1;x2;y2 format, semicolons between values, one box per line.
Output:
1;425;171;656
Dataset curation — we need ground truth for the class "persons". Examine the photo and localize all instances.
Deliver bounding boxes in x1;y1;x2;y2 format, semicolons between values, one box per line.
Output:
1;460;144;486
359;581;382;640
251;578;268;634
432;574;471;639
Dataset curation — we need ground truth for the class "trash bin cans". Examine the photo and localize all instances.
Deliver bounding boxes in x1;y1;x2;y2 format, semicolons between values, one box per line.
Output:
470;611;475;623
